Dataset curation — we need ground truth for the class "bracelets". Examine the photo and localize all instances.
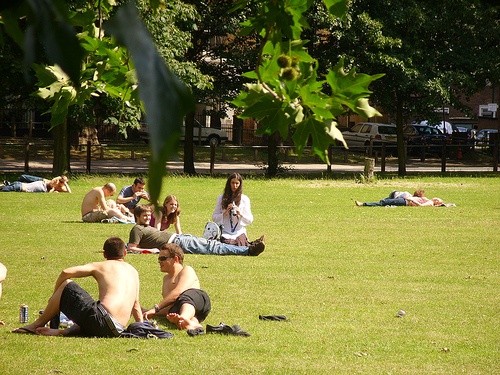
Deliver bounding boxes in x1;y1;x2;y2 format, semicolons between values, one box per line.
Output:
154;304;159;311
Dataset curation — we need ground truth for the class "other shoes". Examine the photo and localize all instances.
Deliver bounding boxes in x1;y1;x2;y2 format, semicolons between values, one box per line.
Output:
187;328;205;335
206;322;225;335
249;235;265;256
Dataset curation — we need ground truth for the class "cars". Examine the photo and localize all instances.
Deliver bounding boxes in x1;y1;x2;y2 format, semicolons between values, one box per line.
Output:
336;123;399;155
138;113;228;148
452;126;470;144
403;124;451;154
476;129;499;143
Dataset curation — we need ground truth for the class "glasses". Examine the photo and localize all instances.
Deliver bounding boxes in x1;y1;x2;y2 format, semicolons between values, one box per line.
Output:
158;256;174;261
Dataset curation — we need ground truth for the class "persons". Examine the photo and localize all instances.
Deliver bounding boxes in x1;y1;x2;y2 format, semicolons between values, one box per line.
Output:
12;237;143;338
150;195;182;234
142;243;211;331
127;205;265;256
116;177;150;218
355;189;445;207
81;182;134;223
0;175;71;193
212;172;254;247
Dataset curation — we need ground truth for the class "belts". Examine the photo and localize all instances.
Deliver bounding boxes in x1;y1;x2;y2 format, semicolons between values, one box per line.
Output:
97;304;119;336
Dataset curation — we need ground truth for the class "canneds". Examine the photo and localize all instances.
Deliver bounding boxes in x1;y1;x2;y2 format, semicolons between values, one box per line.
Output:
19;306;28;323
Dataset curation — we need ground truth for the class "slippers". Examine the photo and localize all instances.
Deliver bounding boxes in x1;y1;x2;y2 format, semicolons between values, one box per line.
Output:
12;328;36;334
